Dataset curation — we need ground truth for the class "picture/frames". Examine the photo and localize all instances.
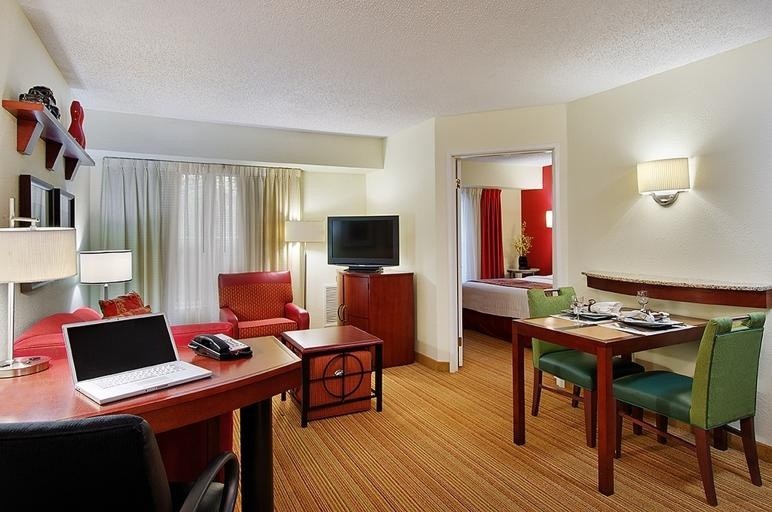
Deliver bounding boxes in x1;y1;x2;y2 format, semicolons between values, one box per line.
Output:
53;187;76;227
18;171;56;293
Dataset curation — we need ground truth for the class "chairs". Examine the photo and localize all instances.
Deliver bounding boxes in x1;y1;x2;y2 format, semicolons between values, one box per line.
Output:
525;288;641;446
216;274;308;341
0;414;242;510
610;308;765;502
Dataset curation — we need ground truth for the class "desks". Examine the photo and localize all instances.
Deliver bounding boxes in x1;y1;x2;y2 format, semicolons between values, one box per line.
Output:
0;332;304;511
509;313;711;494
280;324;384;429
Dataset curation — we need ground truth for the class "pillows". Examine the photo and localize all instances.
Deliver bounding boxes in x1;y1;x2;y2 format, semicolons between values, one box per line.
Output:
104;305;150;318
100;291;146;315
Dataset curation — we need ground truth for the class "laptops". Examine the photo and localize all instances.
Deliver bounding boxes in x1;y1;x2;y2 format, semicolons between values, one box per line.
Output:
60;313;212;407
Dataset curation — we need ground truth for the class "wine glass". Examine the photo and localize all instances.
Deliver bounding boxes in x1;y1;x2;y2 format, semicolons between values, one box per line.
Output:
636;290;648;315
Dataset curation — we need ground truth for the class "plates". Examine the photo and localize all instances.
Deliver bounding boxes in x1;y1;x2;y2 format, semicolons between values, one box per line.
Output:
563;309;684;330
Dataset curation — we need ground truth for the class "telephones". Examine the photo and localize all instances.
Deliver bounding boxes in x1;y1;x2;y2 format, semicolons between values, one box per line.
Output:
189;333;251;361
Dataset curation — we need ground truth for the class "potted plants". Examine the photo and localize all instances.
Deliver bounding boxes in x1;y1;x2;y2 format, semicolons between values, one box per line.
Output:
512;218;533;268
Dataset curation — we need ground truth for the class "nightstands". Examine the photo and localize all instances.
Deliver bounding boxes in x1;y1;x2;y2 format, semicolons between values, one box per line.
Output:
506;267;538;278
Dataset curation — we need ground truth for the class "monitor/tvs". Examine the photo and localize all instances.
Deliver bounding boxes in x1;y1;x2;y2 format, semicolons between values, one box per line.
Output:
328;215;400;271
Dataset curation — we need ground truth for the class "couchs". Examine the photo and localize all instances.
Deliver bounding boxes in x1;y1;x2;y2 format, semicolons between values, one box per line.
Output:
14;309;234;479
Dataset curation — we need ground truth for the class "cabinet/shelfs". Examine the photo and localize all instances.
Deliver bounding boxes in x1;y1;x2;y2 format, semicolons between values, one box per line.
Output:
335;270;415;372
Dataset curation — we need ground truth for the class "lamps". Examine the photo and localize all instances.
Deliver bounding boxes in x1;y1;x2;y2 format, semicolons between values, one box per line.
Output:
635;157;689;206
285;219;326;308
0;198;78;382
544;209;553;230
79;250;134;296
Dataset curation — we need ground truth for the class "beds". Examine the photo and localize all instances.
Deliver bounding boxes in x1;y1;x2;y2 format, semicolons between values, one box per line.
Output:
461;276;552;347
